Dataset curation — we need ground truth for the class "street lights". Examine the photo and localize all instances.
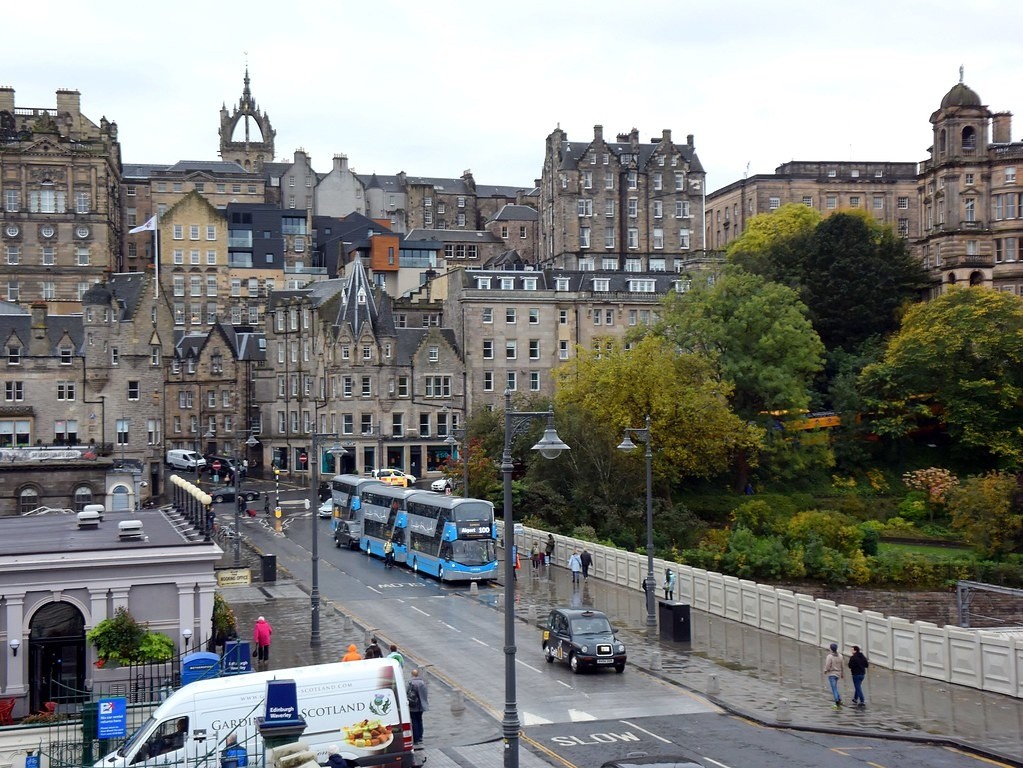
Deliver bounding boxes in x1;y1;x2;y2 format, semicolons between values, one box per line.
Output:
444;421;469;500
309;419;349;649
365;420;382;482
233;423;259;566
502;385;572;768
616;412;658;627
195;426;214;487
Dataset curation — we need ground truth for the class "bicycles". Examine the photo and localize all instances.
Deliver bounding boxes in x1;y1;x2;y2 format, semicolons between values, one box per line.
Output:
210;524;234;542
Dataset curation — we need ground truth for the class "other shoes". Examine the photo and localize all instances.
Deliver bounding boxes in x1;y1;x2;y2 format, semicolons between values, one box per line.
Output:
832;705;841;710
840;701;844;705
858;703;865;706
577;579;579;582
573;580;575;582
535;569;538;573
852;699;858;703
531;568;535;572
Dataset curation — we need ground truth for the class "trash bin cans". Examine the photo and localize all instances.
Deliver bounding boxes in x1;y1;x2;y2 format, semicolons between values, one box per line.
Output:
259;554;277;582
659;600;691;642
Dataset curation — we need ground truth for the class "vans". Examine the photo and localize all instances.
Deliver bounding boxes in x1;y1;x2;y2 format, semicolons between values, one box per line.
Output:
167;449;206;473
207;453;247;478
88;657;428;768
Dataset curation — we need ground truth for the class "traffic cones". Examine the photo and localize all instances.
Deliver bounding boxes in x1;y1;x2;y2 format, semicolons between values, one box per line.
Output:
514;554;522;570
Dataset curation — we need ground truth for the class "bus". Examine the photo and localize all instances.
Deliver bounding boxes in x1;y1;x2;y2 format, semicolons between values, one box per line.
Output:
355;484;504;584
330;474;385;533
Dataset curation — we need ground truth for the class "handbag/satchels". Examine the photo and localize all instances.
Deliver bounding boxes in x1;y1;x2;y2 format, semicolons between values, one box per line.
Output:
516;554;521;569
663;585;669;590
252;643;258;657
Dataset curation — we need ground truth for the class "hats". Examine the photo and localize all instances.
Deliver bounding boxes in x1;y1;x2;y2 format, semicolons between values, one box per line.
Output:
533;540;538;544
258;616;264;621
830;643;837;651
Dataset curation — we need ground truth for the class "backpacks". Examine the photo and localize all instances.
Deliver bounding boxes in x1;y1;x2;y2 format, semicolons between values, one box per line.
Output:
391;654;403;666
407;681;419;709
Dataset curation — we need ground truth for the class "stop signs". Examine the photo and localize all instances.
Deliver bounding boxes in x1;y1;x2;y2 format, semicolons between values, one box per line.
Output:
212;460;221;470
299;453;307;463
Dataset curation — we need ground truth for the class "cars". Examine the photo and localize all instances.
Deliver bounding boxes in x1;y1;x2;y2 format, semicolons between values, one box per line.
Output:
207;485;260;504
430;476;458;492
599;753;705;768
371;469;417;488
318;498;332;520
334;520;362;551
542;607;627;675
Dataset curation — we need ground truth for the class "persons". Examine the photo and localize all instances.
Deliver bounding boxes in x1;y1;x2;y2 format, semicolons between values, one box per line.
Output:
386;644;405;667
824;643;845;711
326;745;347;768
545;534;554;567
208;508;216;529
531;541;540;571
366;638;383;658
159;718;187;753
240;458;257;477
567;551;582;583
271;460;276;480
412;537;422;550
406;670;431;745
384;538;394;568
642;579;648;610
342;645;362;661
580;549;593;582
220;734;249;768
513;566;517;581
224;473;241;488
264;494;271;516
364;649;374;658
238;496;247;517
847;646;870;707
662;568;677;600
253;616;272;662
